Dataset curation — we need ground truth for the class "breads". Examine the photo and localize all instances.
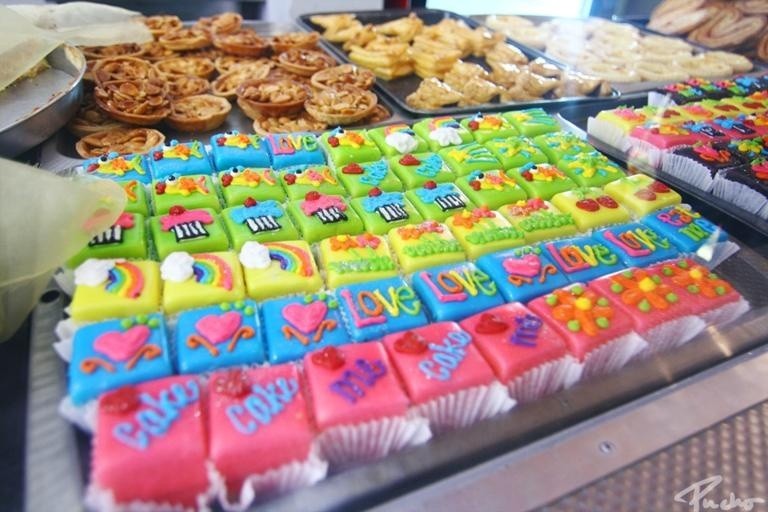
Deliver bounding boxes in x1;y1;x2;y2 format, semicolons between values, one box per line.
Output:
310;0;768;109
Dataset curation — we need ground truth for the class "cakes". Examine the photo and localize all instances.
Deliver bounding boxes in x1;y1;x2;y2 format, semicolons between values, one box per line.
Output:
53;73;768;512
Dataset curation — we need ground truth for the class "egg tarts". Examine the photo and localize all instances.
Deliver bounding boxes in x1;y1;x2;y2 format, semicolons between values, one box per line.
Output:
74;12;391;157
